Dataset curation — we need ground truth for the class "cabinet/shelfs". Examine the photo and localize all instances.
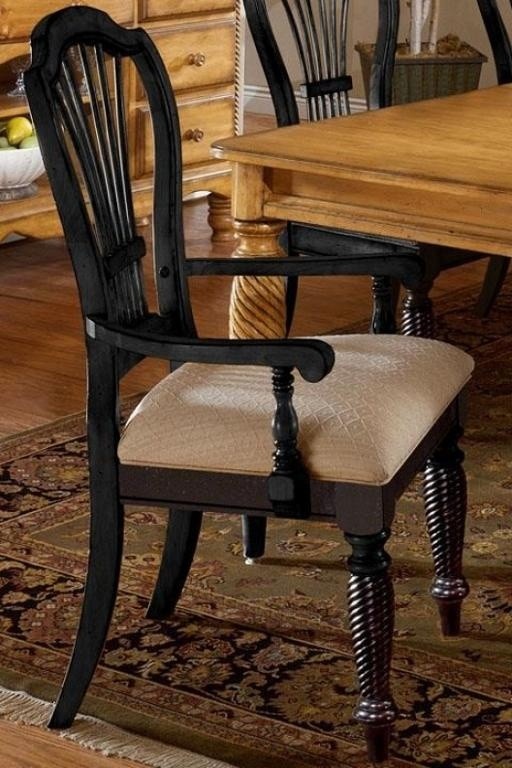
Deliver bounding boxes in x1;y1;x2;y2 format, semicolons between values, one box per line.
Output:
0;0;236;243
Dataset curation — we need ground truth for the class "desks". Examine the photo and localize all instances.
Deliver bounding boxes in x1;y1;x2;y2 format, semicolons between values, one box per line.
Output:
209;84;512;341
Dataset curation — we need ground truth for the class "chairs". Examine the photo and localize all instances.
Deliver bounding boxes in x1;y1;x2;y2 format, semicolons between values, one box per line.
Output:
242;2;502;334
25;8;480;763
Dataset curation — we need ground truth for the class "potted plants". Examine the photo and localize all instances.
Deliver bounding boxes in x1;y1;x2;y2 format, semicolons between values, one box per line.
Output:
352;0;488;109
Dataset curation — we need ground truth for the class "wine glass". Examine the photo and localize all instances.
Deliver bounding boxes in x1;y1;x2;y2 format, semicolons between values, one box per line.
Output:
7;55;32;98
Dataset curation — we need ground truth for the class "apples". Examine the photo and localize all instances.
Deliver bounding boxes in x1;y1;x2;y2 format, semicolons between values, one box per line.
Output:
0;136;38;150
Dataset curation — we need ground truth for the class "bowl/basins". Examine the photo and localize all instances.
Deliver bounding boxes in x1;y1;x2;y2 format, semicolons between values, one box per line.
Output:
0;145;46;202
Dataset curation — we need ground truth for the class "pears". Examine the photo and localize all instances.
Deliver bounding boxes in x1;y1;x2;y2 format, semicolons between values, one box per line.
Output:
7;117;32;144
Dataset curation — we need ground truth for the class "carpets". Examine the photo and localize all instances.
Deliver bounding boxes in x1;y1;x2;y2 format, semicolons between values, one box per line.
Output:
1;270;510;767
